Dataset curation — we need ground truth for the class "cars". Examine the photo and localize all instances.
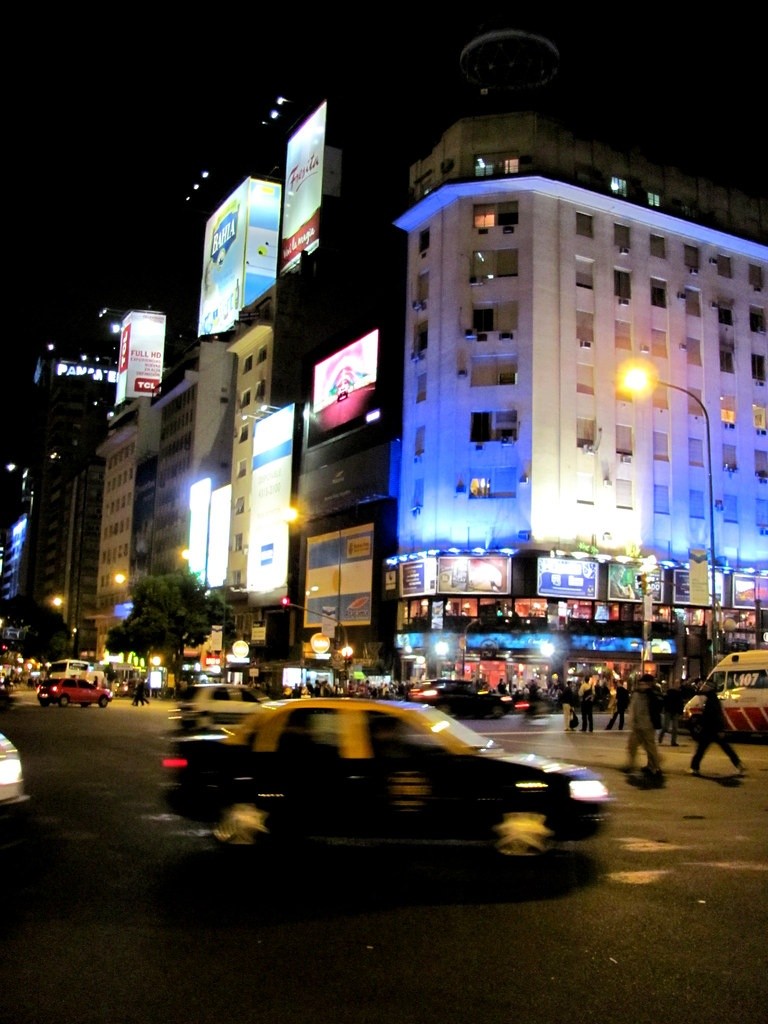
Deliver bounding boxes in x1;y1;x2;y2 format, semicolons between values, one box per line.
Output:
162;696;610;859
169;683;274;729
36;678;113;708
408;678;515;719
110;676;146;698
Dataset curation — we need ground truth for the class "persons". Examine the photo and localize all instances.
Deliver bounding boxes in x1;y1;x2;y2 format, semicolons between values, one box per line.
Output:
3;671;42;692
90;672;149;706
499;669;749;777
174;674;408;698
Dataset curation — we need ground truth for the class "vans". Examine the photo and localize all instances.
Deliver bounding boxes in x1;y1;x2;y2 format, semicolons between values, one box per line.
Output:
683;649;767;743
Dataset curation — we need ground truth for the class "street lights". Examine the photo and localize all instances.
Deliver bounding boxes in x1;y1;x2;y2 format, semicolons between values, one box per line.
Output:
631;641;645;678
622;365;719;688
342;646;354;696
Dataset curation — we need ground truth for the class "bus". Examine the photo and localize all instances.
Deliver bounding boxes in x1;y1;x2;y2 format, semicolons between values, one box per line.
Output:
47;659;90;680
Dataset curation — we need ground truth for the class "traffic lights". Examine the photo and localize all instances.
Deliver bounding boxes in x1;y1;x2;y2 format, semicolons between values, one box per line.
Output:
496;609;504;617
280;598;289;605
